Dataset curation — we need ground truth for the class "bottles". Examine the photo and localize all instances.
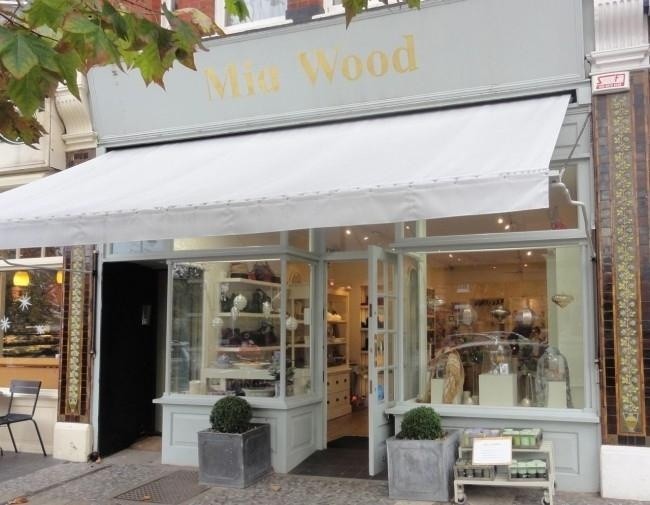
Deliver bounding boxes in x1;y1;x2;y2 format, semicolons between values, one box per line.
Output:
504;428;538;446
457;461;495;480
510;460;547;479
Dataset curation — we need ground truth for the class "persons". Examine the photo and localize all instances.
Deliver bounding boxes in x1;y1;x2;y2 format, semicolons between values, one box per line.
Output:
442;338;466;404
518;327;548;372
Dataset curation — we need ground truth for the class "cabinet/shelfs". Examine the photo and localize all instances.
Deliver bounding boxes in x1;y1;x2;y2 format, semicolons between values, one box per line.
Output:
187;277;436;420
455;440;556;486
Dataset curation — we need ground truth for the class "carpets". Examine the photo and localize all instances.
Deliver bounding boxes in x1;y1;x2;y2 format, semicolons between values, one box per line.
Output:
289;435;388;481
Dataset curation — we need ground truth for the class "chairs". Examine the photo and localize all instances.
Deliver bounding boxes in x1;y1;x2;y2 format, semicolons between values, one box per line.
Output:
0;379;47;457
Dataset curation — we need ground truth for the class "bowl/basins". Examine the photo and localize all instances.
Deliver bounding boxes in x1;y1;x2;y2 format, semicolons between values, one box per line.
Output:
241;386;275;397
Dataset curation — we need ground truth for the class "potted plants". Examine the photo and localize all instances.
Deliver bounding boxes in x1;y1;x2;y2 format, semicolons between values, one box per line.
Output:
386;405;456;502
195;396;275;489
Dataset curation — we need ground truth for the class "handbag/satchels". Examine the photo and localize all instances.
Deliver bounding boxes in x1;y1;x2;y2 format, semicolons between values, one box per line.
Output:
231;262;273;282
221;324;276;345
252;289;272;312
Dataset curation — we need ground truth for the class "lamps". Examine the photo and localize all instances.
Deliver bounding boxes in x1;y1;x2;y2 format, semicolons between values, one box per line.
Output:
552;246;574;308
13;271;29;286
